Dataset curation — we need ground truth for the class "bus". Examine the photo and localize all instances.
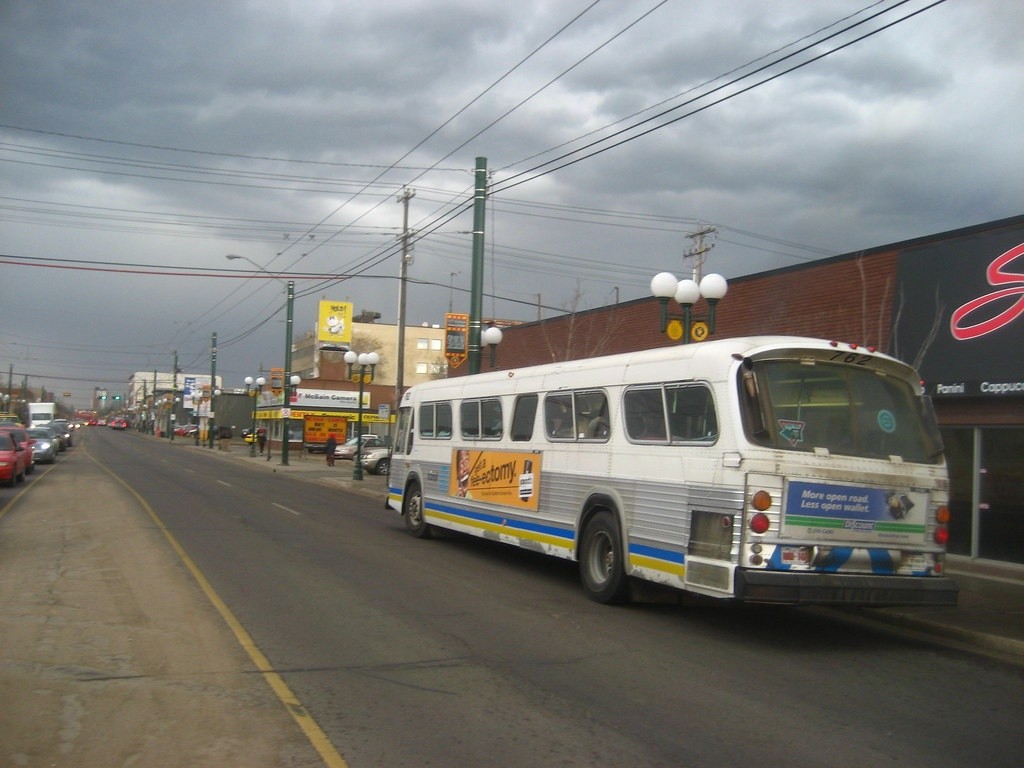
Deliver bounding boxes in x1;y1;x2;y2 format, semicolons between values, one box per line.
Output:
386;335;958;608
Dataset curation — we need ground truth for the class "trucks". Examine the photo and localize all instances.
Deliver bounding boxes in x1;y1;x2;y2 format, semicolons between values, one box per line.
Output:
28;402;56;427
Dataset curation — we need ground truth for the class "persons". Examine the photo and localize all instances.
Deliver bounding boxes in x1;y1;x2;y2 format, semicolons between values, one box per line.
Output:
256;422;267;456
326;433;337;467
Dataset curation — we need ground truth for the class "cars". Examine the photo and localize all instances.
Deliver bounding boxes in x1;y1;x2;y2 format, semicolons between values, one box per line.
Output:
98;419;107;426
303;441;328;453
0;426;36;475
334;434;384;460
0;434;25;486
37;426;60;454
361;446;396;475
246;429;260;445
87;420;96;425
67;421;81;429
174;424;199;438
51;426;72;447
25;428;56;464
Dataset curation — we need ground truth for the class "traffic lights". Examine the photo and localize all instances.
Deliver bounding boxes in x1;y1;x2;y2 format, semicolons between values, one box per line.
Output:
112;396;121;400
98;396;107;399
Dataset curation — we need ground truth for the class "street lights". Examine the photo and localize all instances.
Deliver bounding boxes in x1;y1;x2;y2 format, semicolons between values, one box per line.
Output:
191;391;202;446
281;375;301;466
343;351;379;480
480;322;502;370
245;376;266;457
171;398;180;441
227;255;294;466
651;271;728;344
209;389;221;449
155;398;167;437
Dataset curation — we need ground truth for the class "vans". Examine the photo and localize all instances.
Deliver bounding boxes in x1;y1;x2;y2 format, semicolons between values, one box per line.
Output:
54;419;68;429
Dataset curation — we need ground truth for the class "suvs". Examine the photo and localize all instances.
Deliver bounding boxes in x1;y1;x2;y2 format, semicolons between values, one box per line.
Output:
0;411;24;426
112;419;126;429
